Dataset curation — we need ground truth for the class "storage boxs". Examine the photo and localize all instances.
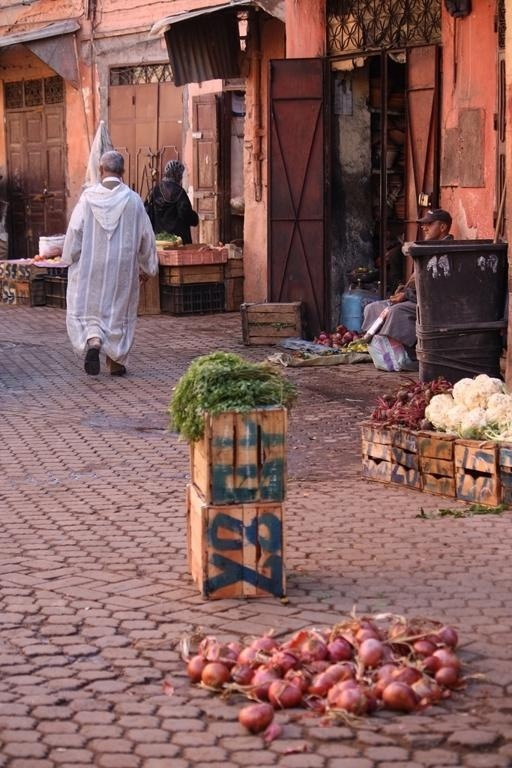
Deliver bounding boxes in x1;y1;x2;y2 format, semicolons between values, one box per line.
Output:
188;405;288;504
240;301;302;348
361;419;512;509
185;483;288;599
136;246;243;315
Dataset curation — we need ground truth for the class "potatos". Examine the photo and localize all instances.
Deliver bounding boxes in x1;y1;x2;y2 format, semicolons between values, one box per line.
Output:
370;378;452;431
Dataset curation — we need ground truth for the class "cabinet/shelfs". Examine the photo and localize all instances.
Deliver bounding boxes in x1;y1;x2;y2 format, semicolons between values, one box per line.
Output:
370;105;404;292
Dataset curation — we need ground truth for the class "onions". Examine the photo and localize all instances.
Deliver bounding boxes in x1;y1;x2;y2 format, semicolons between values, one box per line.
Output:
315;324;364;348
187;616;462;734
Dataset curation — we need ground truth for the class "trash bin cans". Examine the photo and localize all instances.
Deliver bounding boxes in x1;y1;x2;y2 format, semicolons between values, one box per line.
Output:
403;238;504;384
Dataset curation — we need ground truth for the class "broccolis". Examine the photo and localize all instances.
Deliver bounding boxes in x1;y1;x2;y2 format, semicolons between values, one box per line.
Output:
424;374;512;440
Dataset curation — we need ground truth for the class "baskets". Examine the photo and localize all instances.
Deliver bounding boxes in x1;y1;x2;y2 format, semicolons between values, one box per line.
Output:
44;265;69;309
162;282;225;317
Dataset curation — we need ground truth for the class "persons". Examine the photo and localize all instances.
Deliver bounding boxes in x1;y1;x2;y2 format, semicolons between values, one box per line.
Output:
361;208;456;349
146;158;200;248
60;151;163;380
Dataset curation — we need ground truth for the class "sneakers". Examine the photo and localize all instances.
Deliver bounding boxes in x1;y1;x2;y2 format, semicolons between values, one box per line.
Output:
109;362;126;376
84;344;101;376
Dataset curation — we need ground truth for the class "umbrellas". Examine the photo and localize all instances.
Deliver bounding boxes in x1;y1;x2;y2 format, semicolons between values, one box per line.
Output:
79;119;127;198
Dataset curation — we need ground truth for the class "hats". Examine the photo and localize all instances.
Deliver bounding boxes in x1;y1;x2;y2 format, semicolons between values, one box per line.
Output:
415;207;453;225
